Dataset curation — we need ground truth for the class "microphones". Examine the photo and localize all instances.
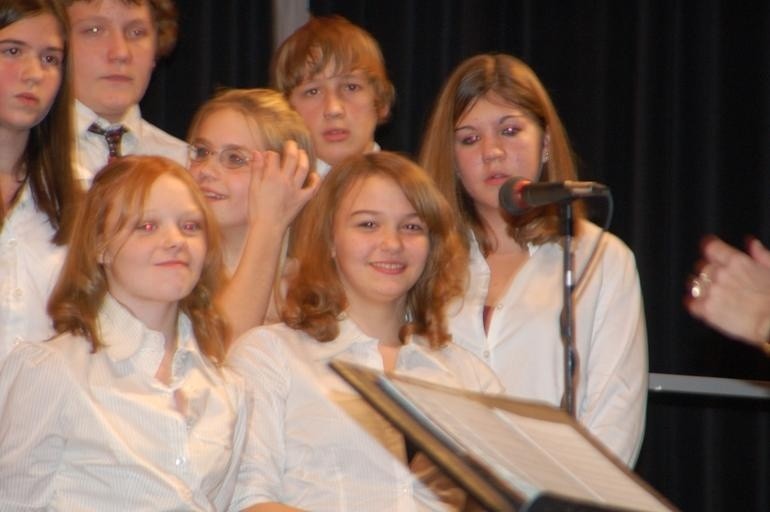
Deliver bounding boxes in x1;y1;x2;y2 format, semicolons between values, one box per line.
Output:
499;175;610;216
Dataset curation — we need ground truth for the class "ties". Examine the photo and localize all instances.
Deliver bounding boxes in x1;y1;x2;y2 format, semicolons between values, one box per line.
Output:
87;122;130;160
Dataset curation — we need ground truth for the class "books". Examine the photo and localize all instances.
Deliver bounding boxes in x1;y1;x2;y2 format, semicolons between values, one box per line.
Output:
376;369;673;511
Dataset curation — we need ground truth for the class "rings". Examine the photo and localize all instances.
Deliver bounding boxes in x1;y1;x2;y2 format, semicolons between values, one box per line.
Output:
689;271;708;300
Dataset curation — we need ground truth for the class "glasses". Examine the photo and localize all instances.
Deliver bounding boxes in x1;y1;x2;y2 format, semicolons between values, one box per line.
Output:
187;141;254;170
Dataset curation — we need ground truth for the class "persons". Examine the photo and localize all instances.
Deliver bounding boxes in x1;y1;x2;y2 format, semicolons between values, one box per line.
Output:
224;151;505;510
1;0;95;369
268;11;395;181
185;88;322;348
678;232;770;356
418;52;650;474
73;1;197;193
0;152;252;510
